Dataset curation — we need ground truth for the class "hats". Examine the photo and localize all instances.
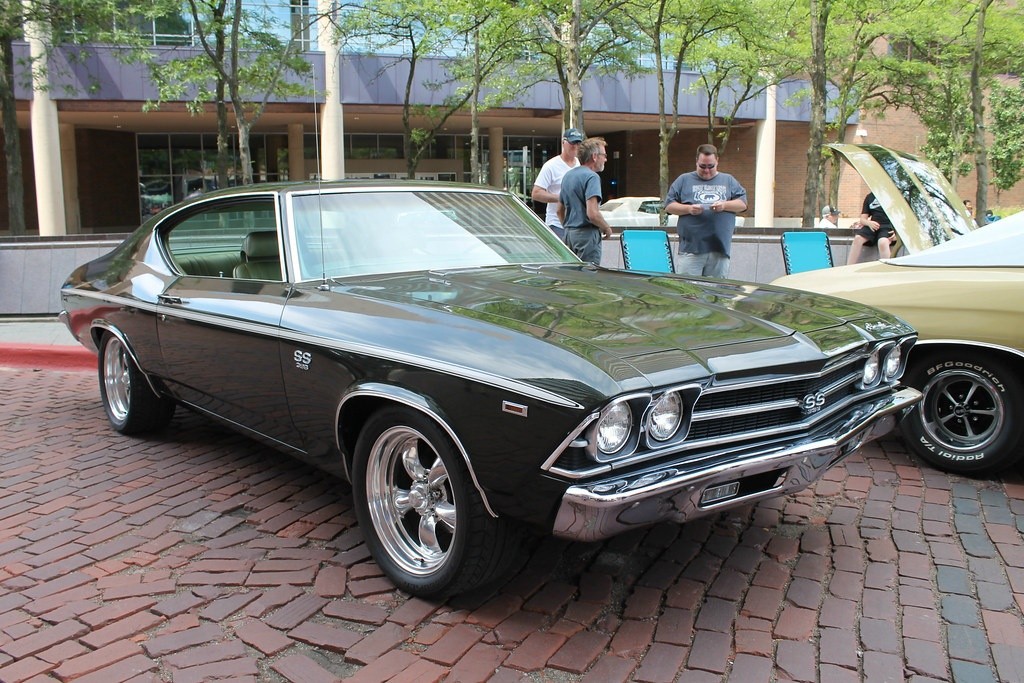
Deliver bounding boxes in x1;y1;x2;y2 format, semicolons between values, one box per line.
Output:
563;129;583;142
822;205;841;216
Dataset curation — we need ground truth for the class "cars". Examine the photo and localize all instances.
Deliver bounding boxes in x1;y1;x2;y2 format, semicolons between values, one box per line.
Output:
598;197;679;220
58;176;925;599
758;141;1024;474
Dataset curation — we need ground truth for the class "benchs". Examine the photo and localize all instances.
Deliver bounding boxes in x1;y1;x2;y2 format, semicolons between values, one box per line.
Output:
175;247;341;278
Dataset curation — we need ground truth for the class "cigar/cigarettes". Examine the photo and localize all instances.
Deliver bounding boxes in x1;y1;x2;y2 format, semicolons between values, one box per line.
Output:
713;206;715;210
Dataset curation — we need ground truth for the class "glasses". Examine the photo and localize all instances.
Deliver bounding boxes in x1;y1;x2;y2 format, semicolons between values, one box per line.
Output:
567;140;581;145
698;163;715;169
597;153;607;157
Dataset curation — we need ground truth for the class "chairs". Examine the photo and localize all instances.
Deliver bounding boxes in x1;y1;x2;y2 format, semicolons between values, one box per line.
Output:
231;232;282;281
780;231;834;276
620;229;676;273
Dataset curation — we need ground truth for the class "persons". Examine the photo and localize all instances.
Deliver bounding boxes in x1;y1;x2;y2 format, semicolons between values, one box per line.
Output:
556;137;612;267
663;144;748;280
963;200;973;216
818;205;841;228
531;128;584;241
846;184;920;265
